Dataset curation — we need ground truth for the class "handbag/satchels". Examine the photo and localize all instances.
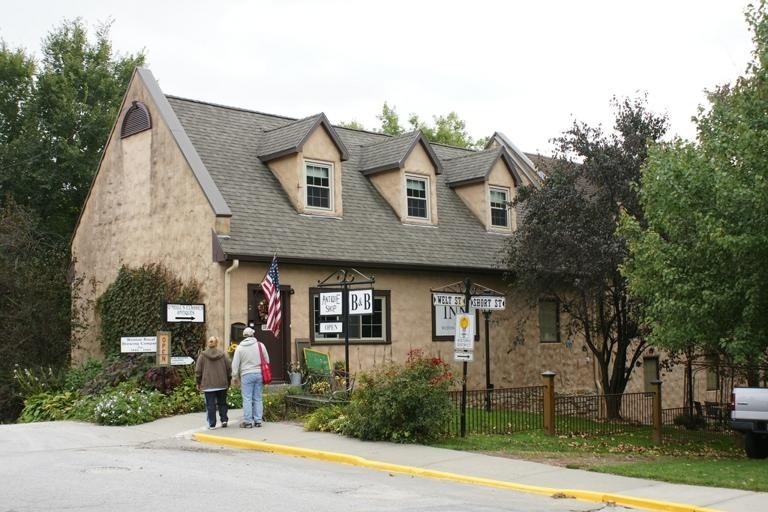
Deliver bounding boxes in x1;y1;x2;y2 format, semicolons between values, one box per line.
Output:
259;342;272;385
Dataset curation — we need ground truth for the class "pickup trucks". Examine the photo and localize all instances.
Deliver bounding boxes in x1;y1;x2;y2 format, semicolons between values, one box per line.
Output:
729;385;768;459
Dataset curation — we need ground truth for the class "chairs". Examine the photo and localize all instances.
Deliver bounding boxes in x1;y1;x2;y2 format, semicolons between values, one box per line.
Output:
691;399;724;431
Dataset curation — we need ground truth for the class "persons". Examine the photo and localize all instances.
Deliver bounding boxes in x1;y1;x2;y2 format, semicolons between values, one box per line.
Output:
231;327;271;428
194;337;232;430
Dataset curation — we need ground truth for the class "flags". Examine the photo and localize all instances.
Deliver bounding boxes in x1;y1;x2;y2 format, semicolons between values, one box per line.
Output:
260;255;282;340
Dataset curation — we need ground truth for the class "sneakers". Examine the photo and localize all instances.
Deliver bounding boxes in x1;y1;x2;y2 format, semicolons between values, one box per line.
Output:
210;425;215;429
241;423;252;428
255;423;261;427
222;421;227;427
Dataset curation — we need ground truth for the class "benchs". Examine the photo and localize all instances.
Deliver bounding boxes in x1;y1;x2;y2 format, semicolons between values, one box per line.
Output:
281;369;356;423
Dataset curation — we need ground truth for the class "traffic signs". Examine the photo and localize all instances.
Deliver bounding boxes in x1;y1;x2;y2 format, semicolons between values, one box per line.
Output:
166;301;204;324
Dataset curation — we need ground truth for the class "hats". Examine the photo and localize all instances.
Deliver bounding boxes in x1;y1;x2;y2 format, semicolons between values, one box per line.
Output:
243;328;255;337
208;337;216;346
